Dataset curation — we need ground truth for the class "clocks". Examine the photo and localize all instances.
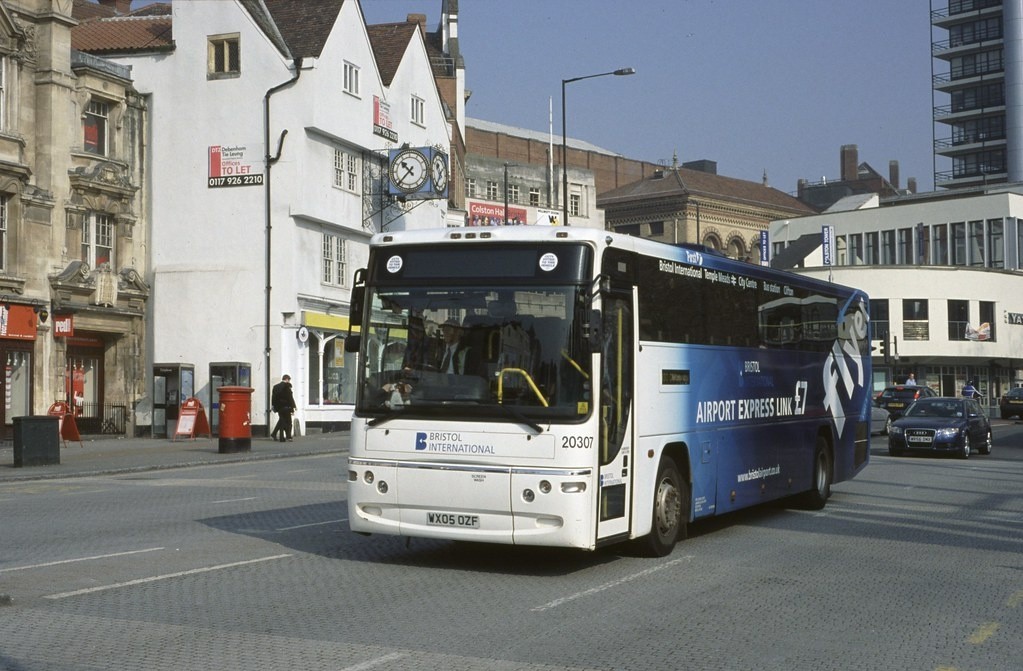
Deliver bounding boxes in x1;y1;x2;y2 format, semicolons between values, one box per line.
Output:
390;150;428;193
431;148;449;198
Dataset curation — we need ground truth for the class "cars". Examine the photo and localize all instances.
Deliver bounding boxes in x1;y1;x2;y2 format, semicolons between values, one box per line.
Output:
875;385;939;420
871;398;891;435
1000;388;1023;419
888;397;993;458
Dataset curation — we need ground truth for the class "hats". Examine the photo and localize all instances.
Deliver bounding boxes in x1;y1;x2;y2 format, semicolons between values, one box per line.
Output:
438;319;469;329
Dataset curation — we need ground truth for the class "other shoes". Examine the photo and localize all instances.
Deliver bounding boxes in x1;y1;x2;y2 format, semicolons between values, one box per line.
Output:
271;433;277;441
280;438;285;442
286;436;293;441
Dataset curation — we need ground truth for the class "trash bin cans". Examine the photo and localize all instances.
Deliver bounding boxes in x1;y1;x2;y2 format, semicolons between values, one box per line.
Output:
11;416;61;466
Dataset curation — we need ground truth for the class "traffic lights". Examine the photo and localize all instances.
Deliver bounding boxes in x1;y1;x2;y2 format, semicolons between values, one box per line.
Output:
880;330;890;363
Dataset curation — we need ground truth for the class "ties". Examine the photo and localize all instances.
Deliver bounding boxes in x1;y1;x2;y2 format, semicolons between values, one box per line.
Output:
440;347;451;373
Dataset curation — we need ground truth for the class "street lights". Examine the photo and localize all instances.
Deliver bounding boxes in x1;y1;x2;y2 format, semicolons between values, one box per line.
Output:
561;67;635;225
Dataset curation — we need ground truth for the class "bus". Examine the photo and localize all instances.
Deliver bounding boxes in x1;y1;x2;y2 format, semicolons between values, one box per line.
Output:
345;224;871;556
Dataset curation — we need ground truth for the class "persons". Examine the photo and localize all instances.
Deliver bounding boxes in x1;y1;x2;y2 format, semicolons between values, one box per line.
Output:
271;375;297;442
906;373;917;385
962;380;983;398
473;215;523;226
432;319;472;375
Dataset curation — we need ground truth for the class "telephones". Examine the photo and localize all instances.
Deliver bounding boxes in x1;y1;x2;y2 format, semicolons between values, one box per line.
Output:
166;389;179;404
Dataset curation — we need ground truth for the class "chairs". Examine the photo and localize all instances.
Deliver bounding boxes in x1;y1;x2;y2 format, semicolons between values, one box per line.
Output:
460;314;751;373
